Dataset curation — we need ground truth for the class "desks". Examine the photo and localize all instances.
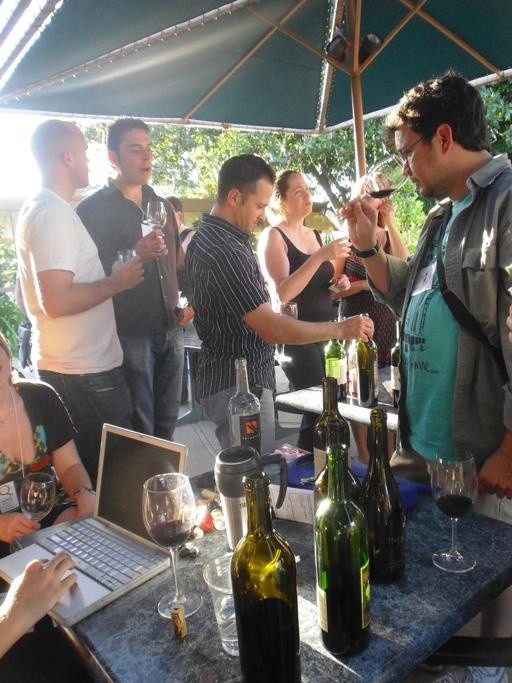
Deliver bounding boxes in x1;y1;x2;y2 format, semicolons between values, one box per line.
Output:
277;363;398;437
12;445;511;683
175;321;206;426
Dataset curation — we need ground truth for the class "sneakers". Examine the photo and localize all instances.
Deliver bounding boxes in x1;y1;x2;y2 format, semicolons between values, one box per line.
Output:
435;665;508;683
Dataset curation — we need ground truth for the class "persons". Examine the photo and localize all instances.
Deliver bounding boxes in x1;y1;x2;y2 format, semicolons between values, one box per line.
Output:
0;329;97;543
0;551;78;663
75;118;185;444
14;118;146;488
166;74;511;637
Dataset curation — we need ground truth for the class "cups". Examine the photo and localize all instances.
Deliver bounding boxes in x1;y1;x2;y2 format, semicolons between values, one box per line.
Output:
116;248;136;265
214;445;288;551
203;552;240;657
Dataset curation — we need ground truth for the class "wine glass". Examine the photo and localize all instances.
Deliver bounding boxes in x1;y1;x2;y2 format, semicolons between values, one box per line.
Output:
20;472;55;523
332;154;410;229
147;202;168;254
142;473;203;619
431;449;478;574
273;302;298;362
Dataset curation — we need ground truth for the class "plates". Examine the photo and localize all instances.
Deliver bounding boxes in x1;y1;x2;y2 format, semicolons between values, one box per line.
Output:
269;482;314;525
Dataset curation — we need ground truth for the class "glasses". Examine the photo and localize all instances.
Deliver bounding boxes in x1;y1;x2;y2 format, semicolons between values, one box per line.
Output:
396;135;424;164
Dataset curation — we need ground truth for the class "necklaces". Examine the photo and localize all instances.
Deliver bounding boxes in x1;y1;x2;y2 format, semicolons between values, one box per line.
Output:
0;388;24;478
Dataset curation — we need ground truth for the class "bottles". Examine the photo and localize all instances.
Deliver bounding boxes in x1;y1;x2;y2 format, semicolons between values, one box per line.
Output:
230;472;303;683
355;314;381;408
230;357;262;457
313;420;367;511
312;378;353;478
361;423;373;510
342;316;352;352
368;407;403;584
316;443;372;656
324;319;347;402
348;339;359;398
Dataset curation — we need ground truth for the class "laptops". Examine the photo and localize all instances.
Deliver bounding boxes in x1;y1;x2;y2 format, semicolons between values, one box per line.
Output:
0;420;190;630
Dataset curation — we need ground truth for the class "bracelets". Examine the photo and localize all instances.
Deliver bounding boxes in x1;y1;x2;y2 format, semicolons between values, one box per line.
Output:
71;487;97;500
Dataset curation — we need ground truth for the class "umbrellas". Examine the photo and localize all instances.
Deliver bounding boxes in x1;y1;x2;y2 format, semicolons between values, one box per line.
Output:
0;0;512;178
